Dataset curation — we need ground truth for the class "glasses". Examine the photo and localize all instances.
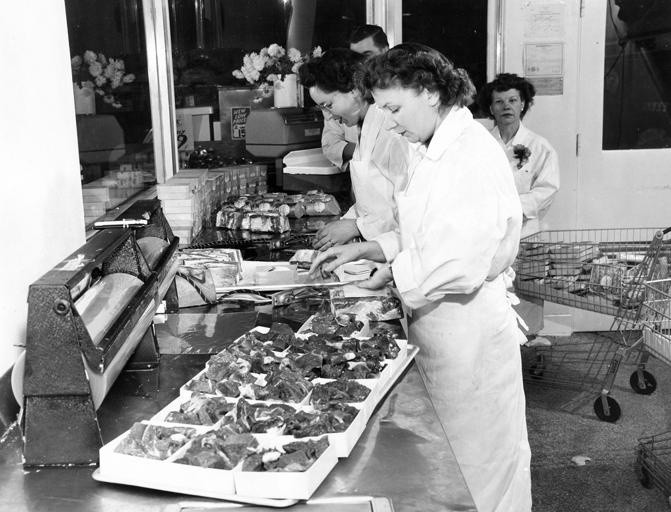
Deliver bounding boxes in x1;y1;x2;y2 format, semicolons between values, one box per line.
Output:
308;93;341;111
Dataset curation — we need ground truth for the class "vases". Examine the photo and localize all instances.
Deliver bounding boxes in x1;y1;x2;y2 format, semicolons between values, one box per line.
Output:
74;81;96;115
274;74;297;108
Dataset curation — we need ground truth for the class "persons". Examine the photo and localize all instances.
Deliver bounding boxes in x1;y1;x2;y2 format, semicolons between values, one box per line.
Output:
303;39;540;512
297;46;420;253
318;21;391;176
479;71;558;347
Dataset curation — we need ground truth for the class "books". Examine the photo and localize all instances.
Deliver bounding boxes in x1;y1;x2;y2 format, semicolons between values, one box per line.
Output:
518;239;601;298
93;197;160;229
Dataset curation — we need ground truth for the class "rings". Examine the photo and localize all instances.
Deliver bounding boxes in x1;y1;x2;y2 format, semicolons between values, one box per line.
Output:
328;241;335;245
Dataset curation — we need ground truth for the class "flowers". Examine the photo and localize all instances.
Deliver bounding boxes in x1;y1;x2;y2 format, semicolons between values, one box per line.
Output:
232;39;323;84
71;52;136;109
